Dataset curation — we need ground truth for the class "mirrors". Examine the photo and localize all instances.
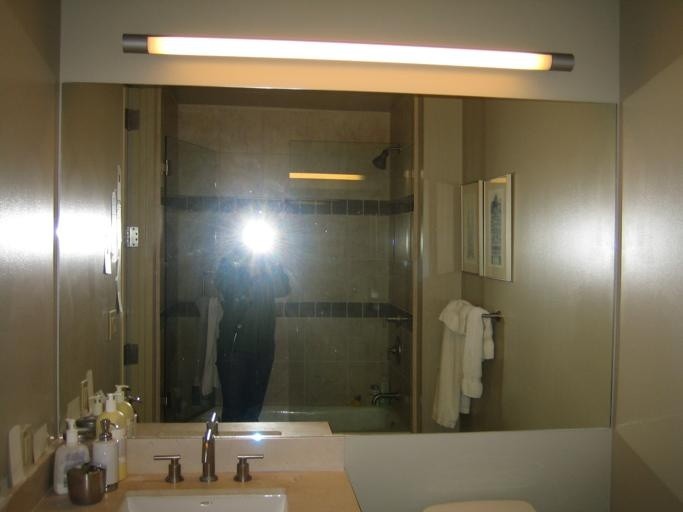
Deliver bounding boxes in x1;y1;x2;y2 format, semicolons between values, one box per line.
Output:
56;82;616;434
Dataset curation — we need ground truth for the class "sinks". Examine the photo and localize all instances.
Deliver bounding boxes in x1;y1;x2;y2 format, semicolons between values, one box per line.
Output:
117;486;288;512
156;430;282;437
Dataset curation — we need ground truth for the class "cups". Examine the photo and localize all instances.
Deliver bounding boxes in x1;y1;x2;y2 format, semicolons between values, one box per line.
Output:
68;462;107;504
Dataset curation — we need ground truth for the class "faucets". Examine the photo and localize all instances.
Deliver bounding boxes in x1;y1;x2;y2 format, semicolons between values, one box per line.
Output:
205;411;219;436
200;427;219;482
372;392;400;407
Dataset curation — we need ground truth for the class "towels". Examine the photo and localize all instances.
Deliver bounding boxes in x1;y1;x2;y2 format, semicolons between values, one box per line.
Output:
432;295;497;428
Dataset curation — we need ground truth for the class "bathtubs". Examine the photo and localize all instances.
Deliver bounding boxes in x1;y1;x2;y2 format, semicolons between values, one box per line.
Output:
186;404;410;432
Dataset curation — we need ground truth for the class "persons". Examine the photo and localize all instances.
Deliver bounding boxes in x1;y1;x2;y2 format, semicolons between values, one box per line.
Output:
215;202;291;422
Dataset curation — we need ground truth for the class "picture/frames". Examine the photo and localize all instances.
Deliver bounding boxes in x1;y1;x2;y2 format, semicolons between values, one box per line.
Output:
458;181;484;275
479;172;512;281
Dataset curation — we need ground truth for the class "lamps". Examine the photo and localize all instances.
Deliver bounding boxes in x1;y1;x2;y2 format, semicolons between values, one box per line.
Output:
373;147;399;168
121;33;575;73
